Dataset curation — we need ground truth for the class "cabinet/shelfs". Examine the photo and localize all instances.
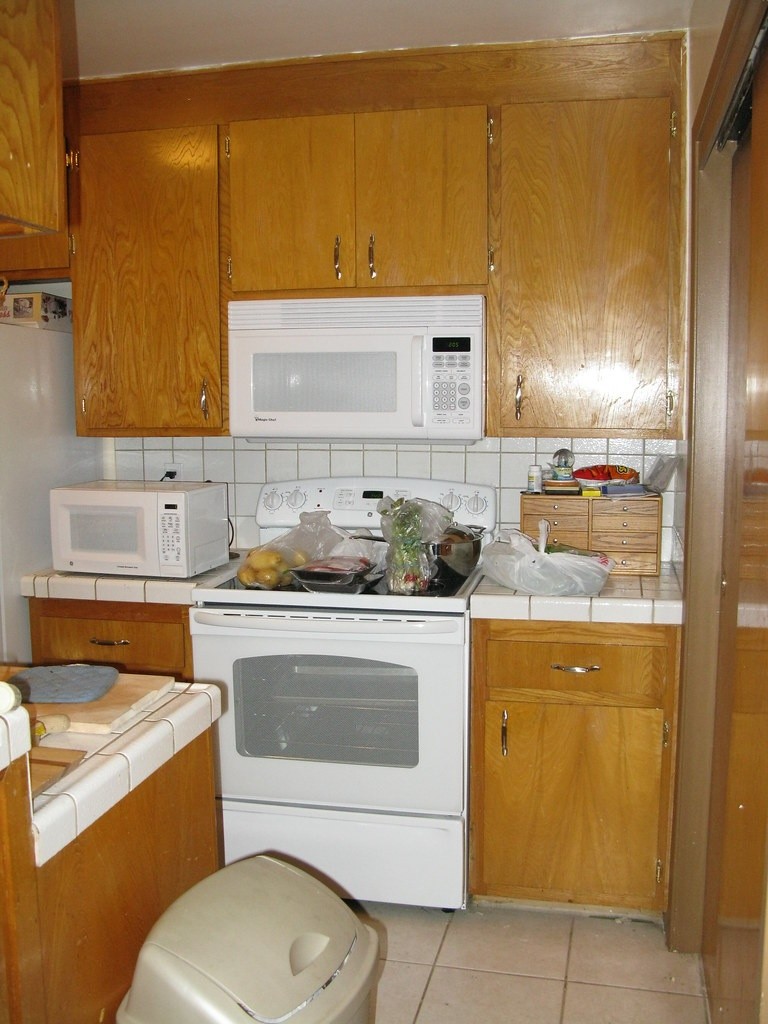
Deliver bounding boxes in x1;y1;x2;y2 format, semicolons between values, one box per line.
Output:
60;63;232;439
520;492;661;578
0;0;71;284
0;663;225;1024
485;29;684;442
19;547;251;680
221;44;493;296
475;561;684;920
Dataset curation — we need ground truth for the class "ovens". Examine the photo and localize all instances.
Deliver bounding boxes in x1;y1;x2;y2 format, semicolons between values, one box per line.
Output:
189;605;471;912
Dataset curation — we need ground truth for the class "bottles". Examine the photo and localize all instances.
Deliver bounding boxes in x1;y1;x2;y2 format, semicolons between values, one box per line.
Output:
527;464;543;493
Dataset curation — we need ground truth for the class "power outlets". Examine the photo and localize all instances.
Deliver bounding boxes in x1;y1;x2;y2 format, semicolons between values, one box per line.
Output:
164;463;184;482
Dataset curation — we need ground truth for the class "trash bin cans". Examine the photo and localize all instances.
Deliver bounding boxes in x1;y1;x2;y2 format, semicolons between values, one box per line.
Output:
115;851;381;1024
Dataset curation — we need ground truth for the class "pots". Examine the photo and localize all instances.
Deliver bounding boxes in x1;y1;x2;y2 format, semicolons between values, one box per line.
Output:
421;520;487;586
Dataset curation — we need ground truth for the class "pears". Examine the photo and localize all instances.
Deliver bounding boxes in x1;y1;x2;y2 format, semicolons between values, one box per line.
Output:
236;548;309;588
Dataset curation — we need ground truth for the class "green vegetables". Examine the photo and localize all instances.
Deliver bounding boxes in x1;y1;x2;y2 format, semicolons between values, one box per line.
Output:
380;497;430;594
532;542;599;556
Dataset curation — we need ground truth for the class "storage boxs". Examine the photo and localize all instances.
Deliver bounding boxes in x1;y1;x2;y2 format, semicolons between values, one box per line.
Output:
0;291;71;328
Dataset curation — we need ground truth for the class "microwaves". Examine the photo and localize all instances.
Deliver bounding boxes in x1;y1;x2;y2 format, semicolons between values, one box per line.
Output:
227;295;487;445
49;480;230;579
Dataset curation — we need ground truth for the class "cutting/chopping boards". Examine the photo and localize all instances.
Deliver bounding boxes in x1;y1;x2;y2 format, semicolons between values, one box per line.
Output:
0;665;175;735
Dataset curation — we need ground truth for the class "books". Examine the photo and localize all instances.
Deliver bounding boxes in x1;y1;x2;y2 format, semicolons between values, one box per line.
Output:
601;484;658;498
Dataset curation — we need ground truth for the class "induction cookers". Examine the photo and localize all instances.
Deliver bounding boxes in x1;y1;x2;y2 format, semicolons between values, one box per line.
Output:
191;476;498;614
